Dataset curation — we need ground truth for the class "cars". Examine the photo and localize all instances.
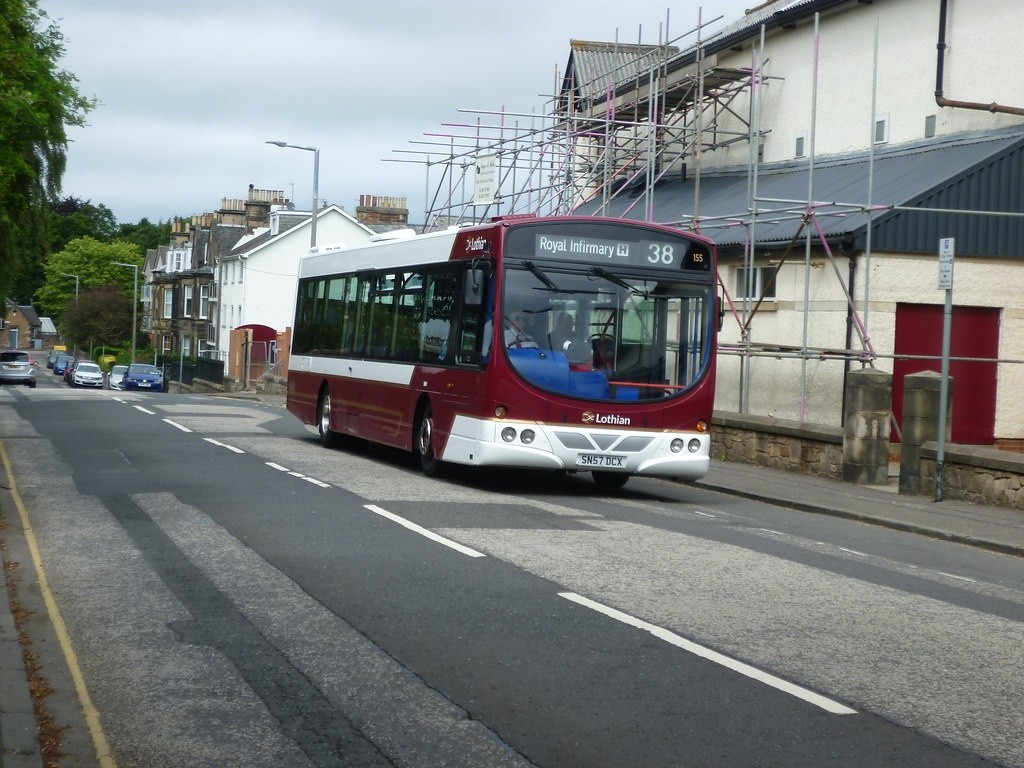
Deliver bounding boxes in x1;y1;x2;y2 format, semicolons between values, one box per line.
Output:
72;363;104;389
47;350;67;369
122;363;163;392
64;359;94;386
53;355;76;375
0;350;38;388
107;365;129;391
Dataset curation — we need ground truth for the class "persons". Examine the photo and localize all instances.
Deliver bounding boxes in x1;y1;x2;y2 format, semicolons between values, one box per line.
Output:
504;309;540;349
548;312;577;351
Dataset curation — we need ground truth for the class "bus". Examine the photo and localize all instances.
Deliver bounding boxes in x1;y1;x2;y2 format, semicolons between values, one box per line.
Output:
286;215;725;491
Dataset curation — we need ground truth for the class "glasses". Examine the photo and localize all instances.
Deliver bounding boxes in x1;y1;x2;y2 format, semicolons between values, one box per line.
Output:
515;316;528;322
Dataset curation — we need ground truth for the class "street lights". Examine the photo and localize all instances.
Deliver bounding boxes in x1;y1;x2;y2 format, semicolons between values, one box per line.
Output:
59;271;78;306
110;261;138;363
266;141;321;250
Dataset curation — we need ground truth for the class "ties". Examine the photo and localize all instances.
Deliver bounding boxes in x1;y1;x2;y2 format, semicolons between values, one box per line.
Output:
514;335;521;347
